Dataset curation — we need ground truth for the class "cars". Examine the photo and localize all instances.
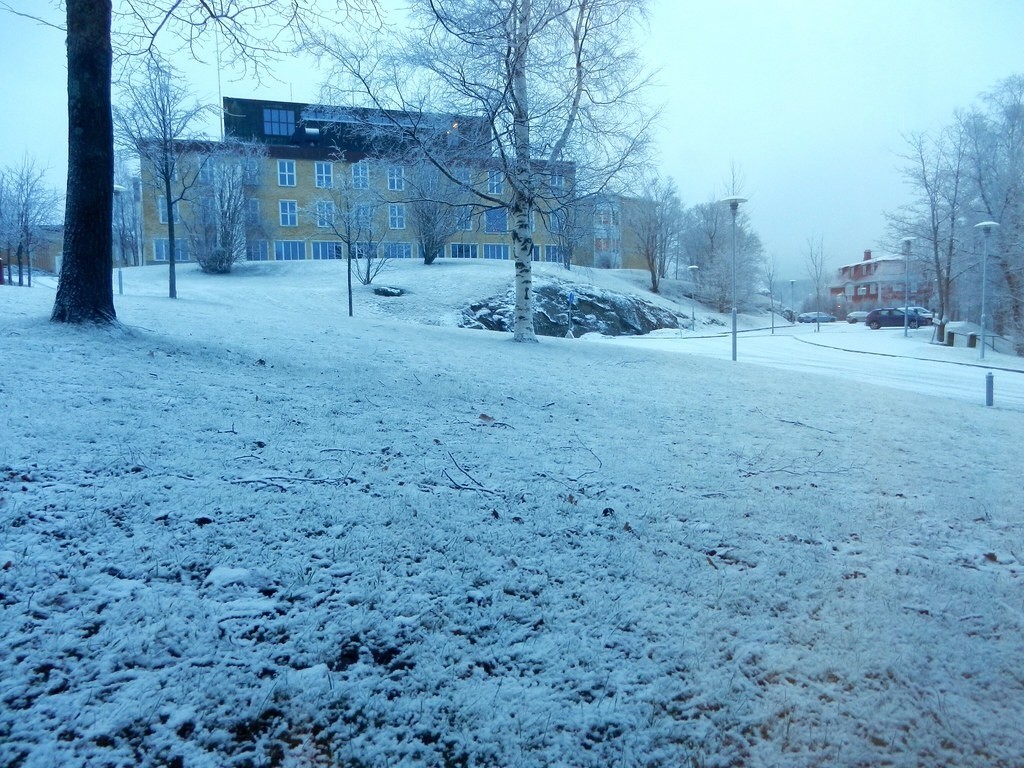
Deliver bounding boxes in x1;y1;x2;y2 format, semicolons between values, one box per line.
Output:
866;306;940;330
798;312;838;323
846;312;869;324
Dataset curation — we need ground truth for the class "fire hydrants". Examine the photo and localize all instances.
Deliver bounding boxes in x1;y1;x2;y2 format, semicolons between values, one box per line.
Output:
985;371;993;405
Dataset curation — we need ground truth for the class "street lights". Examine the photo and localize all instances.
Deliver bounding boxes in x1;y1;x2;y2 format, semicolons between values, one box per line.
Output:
112;184;126;295
899;234;918;336
788;280;796;324
720;195;749;363
975;221;1002;360
688;263;701;336
